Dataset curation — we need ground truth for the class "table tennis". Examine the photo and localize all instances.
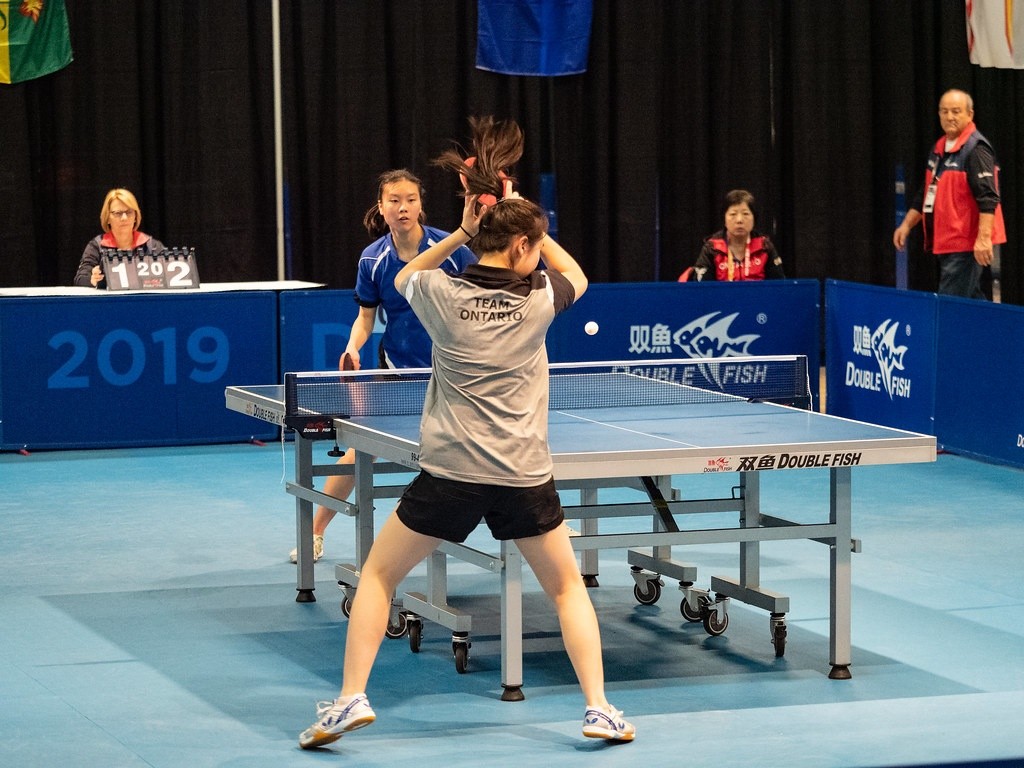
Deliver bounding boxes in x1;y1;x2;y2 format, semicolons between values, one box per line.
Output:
585;322;599;335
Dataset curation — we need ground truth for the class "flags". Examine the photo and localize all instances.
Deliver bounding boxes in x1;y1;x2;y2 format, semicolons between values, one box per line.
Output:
0;0;74;83
965;0;1024;70
476;0;593;76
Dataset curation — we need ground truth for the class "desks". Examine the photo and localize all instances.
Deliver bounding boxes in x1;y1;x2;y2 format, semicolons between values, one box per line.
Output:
224;373;945;701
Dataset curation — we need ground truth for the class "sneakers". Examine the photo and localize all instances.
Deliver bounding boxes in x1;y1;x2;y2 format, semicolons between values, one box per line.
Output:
298;693;375;747
565;526;582;536
290;535;324;562
583;707;636;739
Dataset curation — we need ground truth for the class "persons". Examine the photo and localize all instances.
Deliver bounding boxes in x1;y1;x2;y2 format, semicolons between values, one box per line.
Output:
73;187;164;287
286;168;479;566
298;120;641;746
676;187;790;283
893;89;1009;303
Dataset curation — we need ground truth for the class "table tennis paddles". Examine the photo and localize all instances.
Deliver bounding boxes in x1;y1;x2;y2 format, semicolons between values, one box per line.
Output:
458;157;509;206
339;352;362;383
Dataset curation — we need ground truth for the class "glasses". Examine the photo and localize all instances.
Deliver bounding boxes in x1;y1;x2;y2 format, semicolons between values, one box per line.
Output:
110;209;134;217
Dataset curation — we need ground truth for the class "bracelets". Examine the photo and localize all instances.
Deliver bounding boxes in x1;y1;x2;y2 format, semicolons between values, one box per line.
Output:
459;225;473;241
